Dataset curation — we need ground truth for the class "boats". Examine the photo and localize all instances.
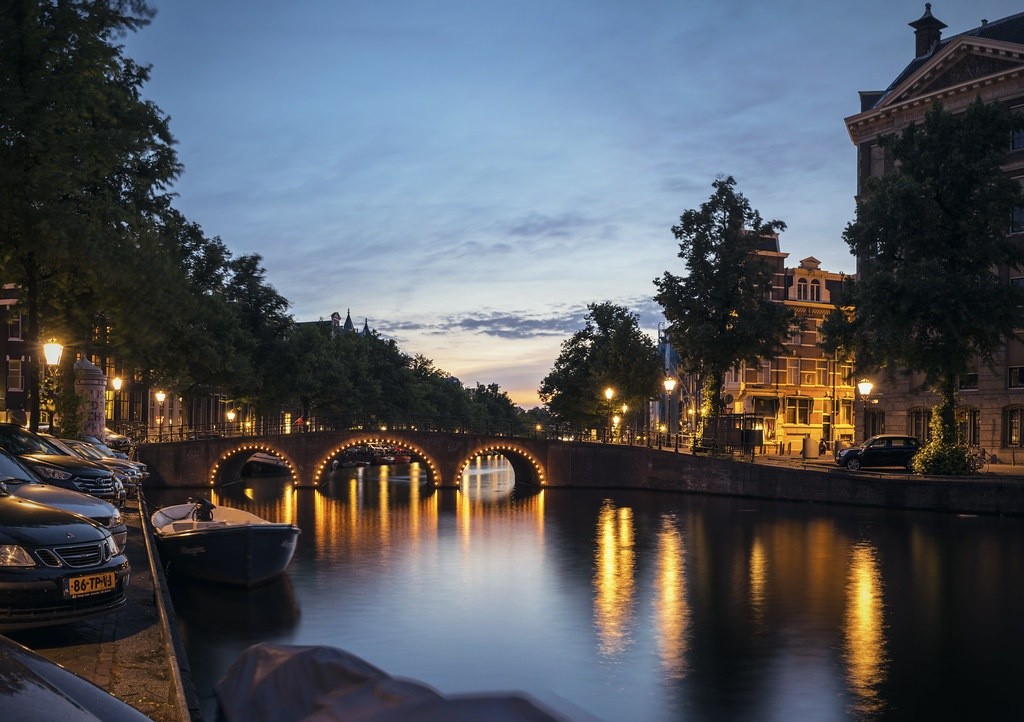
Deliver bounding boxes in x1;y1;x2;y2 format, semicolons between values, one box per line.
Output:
342;460;371;467
384;448;412;463
375;456;396;464
151;495;300;584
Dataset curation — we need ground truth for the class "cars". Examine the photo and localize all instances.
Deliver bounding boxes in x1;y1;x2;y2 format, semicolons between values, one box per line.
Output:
39;432;137;495
64;434;118;458
0;447;128;552
61;438;143;486
0;423;126;510
0;481;131;632
834;434;923;471
111;449;150;478
0;635;156;722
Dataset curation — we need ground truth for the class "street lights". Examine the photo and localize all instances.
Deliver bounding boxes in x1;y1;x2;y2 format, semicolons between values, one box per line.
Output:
663;375;677;447
856;378;874;441
155;390;166;441
620;403;628;444
113;377;121;432
606;388;613;443
42;334;63;436
227;412;234;436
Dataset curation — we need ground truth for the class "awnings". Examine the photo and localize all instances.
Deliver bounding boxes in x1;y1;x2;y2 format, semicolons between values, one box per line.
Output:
783;393;815;415
751;394;781;420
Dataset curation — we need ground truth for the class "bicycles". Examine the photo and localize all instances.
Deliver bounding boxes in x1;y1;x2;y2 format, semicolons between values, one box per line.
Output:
968;453;989;475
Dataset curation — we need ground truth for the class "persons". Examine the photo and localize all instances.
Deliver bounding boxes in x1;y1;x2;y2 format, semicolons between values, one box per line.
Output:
292;415;305;433
